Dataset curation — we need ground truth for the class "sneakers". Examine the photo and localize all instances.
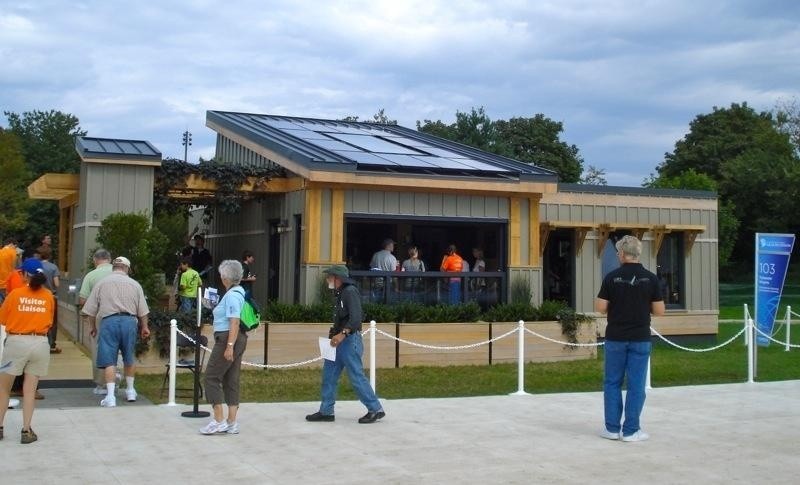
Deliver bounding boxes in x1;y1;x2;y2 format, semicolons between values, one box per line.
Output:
623;430;650;442
18;389;44;400
20;428;37;445
199;419;229;435
224;421;240;435
101;396;116;408
93;387;108;395
125;389;137;402
599;429;620;440
51;342;56;348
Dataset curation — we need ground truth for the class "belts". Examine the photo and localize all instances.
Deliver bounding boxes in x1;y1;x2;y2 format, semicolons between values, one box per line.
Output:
113;312;136;316
9;331;46;337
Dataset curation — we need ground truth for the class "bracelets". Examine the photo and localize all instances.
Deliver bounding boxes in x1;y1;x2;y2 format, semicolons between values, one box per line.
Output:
227;343;234;346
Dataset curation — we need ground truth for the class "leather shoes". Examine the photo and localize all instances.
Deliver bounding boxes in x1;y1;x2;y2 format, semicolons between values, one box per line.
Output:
306;411;335;422
358;411;385;423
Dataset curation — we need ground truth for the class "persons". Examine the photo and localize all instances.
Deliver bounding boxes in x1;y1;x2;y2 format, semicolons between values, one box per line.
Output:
594;234;665;442
306;266;385;425
0;231;63;446
198;259;248;435
400;246;426;304
80;248;121;396
238;249;258;313
469;247;486;293
440;244;463;305
176;257;202;316
182;224;213;296
82;256;150;408
369;239;399;303
173;246;199;313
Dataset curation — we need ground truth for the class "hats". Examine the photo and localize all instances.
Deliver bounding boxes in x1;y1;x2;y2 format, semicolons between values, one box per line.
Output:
321;264;357;285
384;238;396;245
115;257;131;268
16;258;43;275
194;233;206;240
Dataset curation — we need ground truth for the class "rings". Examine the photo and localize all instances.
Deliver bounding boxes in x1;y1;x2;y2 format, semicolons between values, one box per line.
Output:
335;342;337;344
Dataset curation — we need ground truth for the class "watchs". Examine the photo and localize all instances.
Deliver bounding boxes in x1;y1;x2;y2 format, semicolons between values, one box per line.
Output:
341;329;347;337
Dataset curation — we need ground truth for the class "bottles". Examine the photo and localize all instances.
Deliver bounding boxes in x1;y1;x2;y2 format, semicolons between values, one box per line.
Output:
396;259;400;272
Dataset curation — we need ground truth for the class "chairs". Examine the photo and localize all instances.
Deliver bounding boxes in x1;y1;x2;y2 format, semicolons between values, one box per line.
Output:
159;336;207;402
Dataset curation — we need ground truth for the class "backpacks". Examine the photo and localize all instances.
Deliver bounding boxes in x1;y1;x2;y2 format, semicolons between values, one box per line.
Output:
234;289;262;333
